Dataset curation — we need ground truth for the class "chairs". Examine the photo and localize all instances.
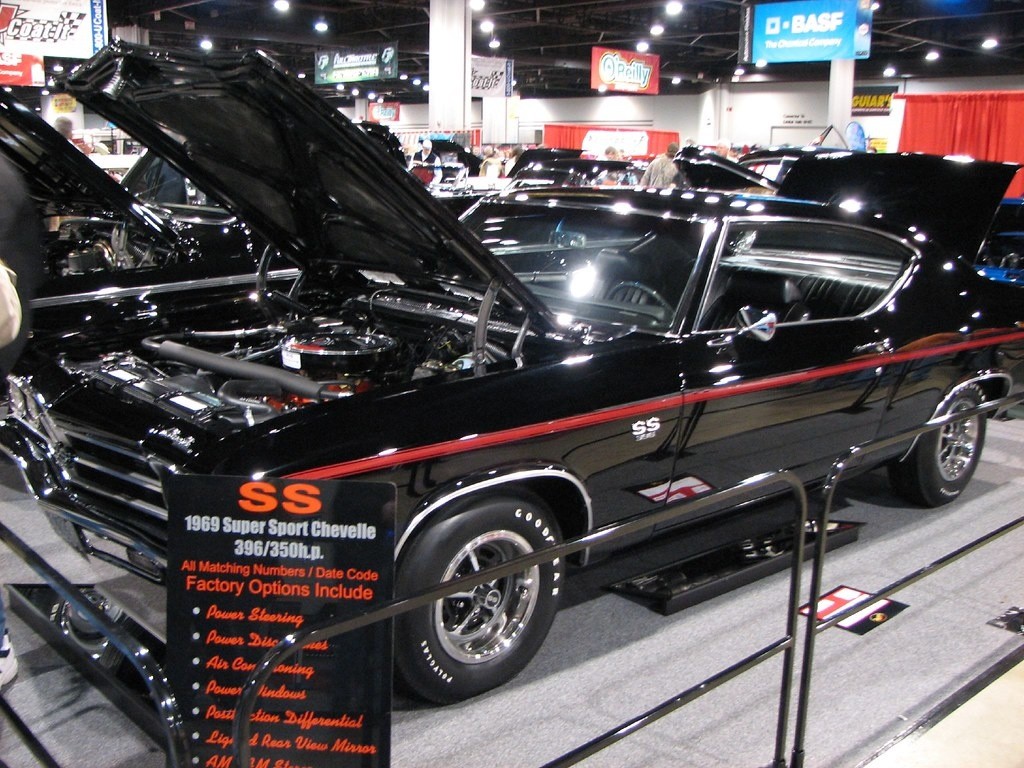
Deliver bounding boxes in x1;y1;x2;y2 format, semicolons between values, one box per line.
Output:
696;267;776;333
592;247;660;306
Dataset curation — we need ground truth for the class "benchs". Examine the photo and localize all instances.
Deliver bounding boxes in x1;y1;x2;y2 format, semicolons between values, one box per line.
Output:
682;258;892;322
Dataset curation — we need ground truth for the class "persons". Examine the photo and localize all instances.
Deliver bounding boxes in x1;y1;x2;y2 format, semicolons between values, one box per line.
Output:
505;144;524;177
716;139;738;163
639;142;679;187
0;259;22;685
479;145;501;178
408;139;442;184
606;146;619;161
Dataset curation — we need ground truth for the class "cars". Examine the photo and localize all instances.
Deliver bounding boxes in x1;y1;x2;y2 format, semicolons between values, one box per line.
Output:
0;86;407;298
409;139;1024;284
0;39;1024;705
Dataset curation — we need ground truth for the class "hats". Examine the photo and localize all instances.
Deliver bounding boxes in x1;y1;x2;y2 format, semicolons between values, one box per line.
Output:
423;140;432;148
667;143;679;154
